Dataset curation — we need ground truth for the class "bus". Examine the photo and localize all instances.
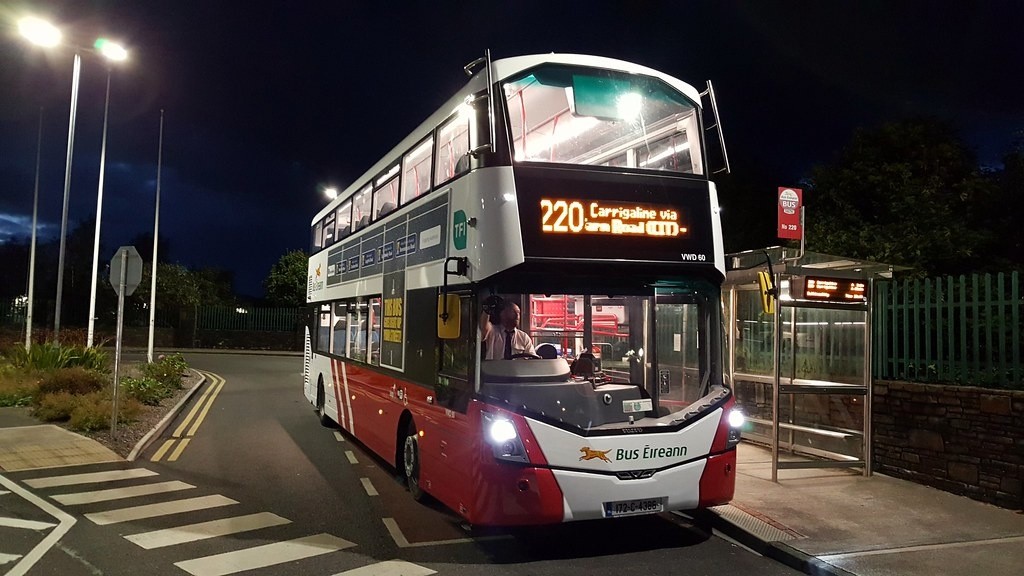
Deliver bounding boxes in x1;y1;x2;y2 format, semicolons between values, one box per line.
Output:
302;52;743;527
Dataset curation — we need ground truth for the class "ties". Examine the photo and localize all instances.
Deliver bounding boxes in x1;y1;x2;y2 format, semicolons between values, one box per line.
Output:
504;330;515;360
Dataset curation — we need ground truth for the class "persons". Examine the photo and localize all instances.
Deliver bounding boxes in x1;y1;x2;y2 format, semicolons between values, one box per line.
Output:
479;301;543;360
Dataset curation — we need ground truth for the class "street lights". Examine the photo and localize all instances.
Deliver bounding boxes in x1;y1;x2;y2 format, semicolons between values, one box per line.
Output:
13;12;65;353
84;36;132;346
